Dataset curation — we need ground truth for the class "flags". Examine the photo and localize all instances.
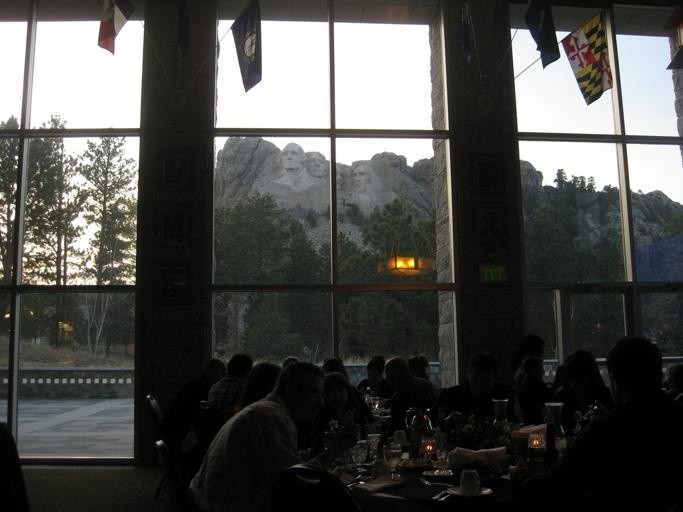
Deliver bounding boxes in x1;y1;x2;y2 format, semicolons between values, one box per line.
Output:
561;11;612;104
98;0;135;55
230;0;261;92
524;0;560;68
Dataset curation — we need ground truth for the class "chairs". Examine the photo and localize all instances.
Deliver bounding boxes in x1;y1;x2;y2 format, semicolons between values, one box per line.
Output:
143;382;683;512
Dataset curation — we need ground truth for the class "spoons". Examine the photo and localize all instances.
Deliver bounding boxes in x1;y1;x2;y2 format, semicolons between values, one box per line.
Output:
419;476;453;487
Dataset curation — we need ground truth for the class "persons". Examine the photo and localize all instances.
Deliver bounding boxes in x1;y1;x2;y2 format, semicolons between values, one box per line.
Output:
156;333;682;510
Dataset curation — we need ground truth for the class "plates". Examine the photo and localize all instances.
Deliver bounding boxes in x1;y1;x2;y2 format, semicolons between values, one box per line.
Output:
447;488;493;497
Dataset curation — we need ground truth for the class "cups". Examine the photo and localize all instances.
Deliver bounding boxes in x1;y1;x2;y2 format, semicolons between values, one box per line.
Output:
460;469;482;494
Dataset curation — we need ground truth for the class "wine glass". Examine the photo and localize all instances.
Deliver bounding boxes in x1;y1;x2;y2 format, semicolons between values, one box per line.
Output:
383;443;401;481
351;442;368;477
